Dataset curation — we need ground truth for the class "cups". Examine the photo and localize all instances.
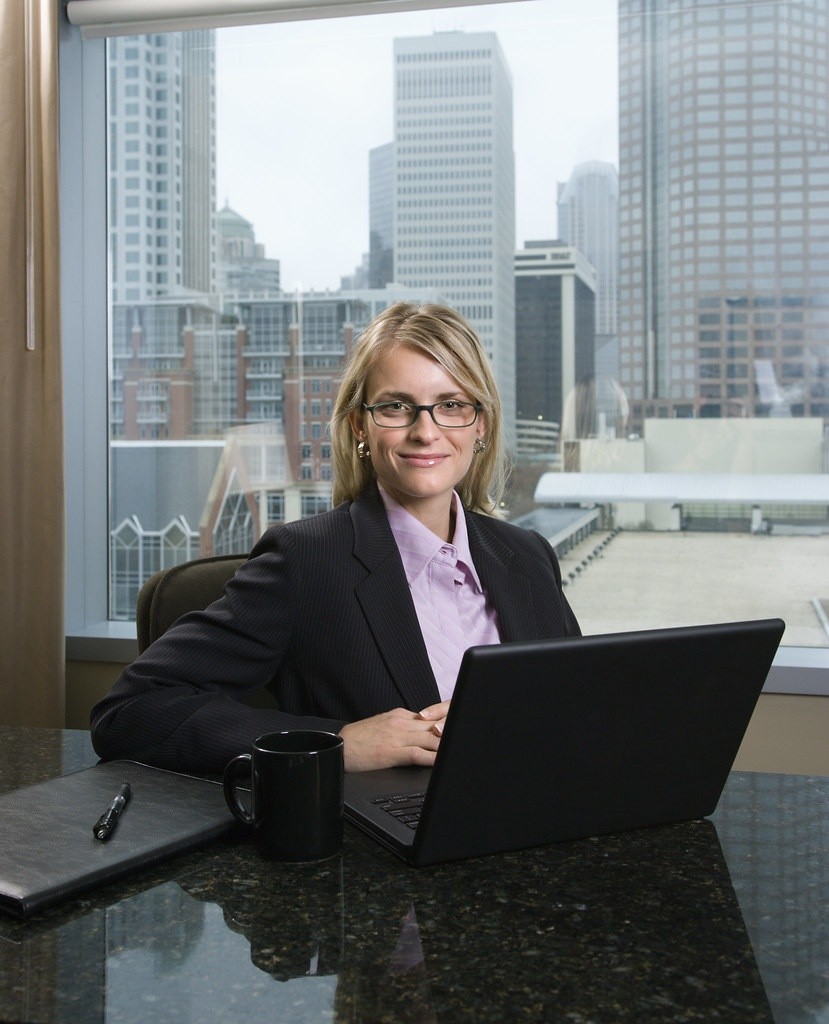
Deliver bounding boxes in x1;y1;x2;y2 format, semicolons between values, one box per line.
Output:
223;730;344;864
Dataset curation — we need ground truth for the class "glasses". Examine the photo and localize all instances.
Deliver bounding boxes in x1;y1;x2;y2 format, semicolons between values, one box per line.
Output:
362;401;481;427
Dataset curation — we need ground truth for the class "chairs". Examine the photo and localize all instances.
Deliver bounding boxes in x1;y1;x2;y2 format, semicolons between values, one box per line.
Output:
135;555;250;655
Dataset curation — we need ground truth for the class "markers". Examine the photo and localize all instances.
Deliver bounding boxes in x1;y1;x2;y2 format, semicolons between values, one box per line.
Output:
92;783;131;840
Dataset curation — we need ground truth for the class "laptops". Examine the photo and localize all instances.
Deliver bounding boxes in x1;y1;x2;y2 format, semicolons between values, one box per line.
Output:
340;619;785;867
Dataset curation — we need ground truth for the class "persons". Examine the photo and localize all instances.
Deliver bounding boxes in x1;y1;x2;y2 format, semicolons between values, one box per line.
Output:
89;303;582;772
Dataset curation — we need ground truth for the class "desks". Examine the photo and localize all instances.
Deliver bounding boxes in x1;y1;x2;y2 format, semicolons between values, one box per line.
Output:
0;726;828;1024
